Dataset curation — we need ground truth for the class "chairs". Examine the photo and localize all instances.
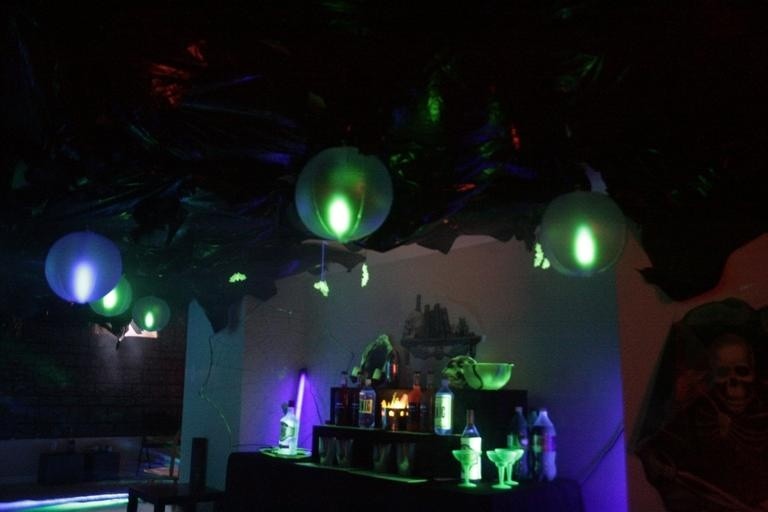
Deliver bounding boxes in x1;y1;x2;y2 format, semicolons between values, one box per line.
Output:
136;414;182;476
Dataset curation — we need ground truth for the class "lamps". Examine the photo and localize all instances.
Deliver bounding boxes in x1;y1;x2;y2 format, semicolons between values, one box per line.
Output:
132;296;170;331
295;144;396;246
540;175;627;276
45;229;123;304
88;275;134;317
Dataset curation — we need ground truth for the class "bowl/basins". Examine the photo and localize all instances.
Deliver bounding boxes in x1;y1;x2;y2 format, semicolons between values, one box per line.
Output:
398;332;482;373
463;361;515;390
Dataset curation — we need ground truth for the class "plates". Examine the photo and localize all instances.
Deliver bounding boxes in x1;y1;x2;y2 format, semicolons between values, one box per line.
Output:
255;446;313;462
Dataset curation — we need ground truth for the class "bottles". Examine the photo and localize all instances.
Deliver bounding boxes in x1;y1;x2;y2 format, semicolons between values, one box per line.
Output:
398;292;471;338
432;370;454;437
280;397;297;455
461;410;482;486
505;405;563;483
332;368;434;435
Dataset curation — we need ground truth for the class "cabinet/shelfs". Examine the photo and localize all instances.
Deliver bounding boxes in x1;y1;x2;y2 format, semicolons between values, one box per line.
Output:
311;386;528;481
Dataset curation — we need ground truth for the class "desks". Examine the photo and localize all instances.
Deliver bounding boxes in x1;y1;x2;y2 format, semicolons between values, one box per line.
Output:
224;450;586;511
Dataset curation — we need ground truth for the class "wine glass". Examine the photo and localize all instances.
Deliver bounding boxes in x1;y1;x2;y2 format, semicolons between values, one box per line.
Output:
485;448;524;490
453;449;481;489
